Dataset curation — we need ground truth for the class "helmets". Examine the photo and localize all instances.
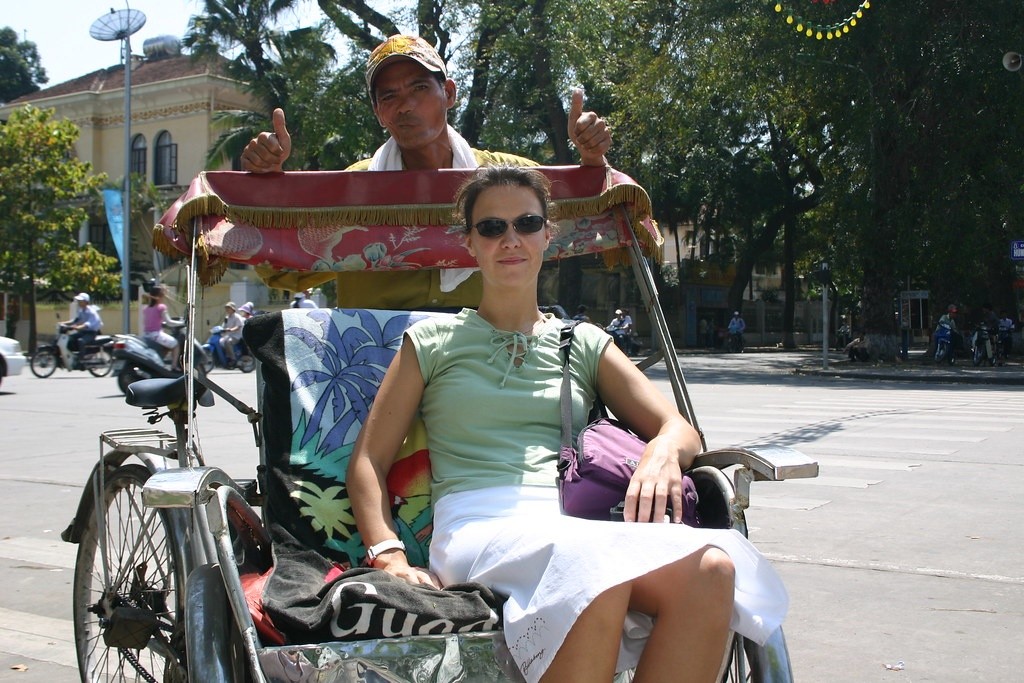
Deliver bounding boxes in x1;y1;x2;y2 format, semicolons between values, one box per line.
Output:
948;308;957;314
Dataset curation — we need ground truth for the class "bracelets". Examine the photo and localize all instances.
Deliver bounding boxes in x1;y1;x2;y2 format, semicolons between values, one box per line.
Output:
363;539;407;568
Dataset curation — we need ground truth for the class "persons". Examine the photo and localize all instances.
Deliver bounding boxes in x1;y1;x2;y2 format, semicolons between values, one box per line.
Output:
837;320;850;346
997;310;1014;358
727;312;746;353
141;286;186;373
981;304;1001;353
842;332;872;360
341;165;791;683
5;304;20;339
241;34;613;313
931;302;959;363
58;291;103;369
697;316;720;347
607;306;634;354
289;287;329;310
220;302;256;366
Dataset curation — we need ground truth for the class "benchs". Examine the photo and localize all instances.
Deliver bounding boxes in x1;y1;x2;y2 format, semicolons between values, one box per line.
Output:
143;303;820;683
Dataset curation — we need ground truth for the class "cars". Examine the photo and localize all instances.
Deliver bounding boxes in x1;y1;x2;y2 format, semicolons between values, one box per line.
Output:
0;335;27;387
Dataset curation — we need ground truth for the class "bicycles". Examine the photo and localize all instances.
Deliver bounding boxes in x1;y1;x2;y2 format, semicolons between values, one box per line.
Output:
726;327;745;354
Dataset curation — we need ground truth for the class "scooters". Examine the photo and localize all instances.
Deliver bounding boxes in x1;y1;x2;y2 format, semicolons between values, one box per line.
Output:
106;314;208;398
932;316;957;365
969;320;1016;367
201;317;259;374
595;319;638;357
30;314;115;379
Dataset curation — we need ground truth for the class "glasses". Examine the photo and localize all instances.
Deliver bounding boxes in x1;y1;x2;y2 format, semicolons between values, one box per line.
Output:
469;214;549;238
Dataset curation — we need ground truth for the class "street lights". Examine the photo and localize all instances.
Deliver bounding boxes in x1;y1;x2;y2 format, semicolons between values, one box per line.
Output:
88;7;151;334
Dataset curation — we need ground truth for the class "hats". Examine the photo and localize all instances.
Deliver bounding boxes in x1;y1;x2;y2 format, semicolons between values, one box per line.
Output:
226;301;238;313
615;309;622;314
239;304;252;315
734;311;740;316
74;292;90;303
366;36;449;114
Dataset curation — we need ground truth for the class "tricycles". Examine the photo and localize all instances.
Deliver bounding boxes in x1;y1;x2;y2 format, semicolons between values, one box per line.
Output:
62;162;824;682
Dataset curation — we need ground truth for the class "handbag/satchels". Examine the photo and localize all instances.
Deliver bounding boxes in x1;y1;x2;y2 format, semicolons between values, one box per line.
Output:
557;320;702;530
